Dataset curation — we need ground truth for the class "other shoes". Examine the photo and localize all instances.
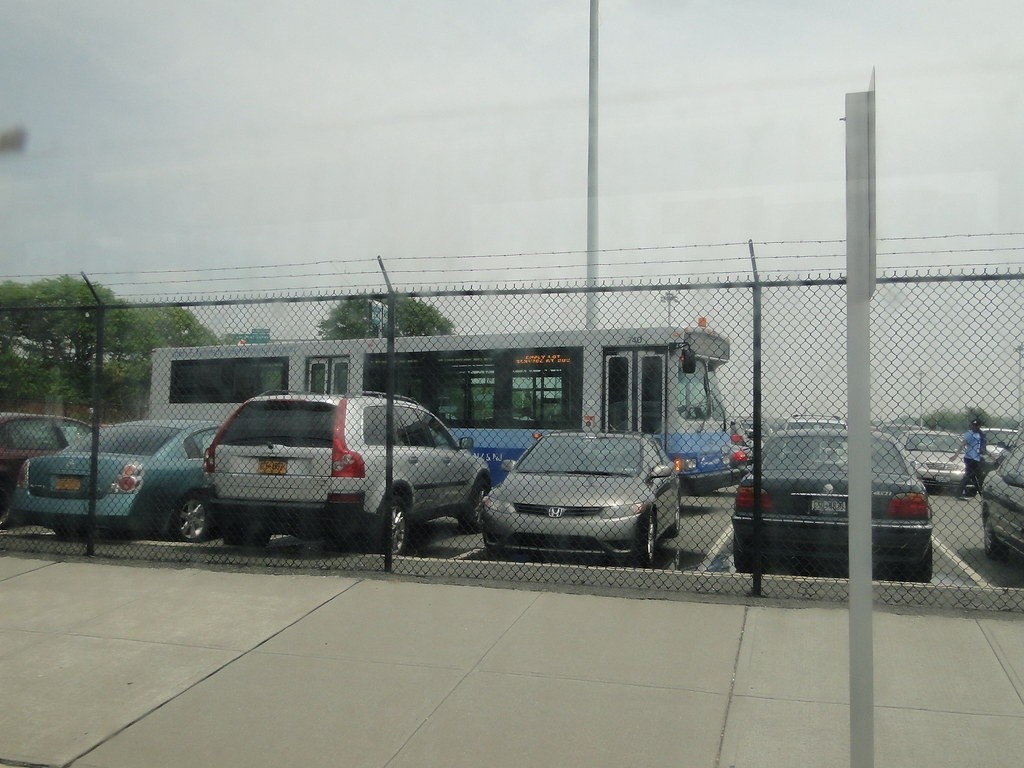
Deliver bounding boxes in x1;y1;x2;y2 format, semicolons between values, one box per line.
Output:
955;495;968;501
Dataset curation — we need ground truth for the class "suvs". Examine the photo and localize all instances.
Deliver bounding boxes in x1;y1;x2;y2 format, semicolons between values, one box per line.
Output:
199;390;491;556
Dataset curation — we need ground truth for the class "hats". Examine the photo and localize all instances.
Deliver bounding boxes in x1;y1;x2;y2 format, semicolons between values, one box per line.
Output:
971;420;982;425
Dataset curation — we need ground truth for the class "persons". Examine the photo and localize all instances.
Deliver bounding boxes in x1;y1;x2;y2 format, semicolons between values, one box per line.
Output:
949;419;985;499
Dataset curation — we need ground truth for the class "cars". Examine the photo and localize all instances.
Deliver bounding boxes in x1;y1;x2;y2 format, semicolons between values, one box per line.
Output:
0;411;102;529
724;415;925;467
731;426;937;583
13;417;222;542
473;430;682;569
978;429;1018;497
977;424;1024;563
898;428;977;499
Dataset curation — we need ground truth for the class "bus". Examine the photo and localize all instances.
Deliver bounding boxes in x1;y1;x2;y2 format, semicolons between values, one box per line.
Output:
147;327;748;493
